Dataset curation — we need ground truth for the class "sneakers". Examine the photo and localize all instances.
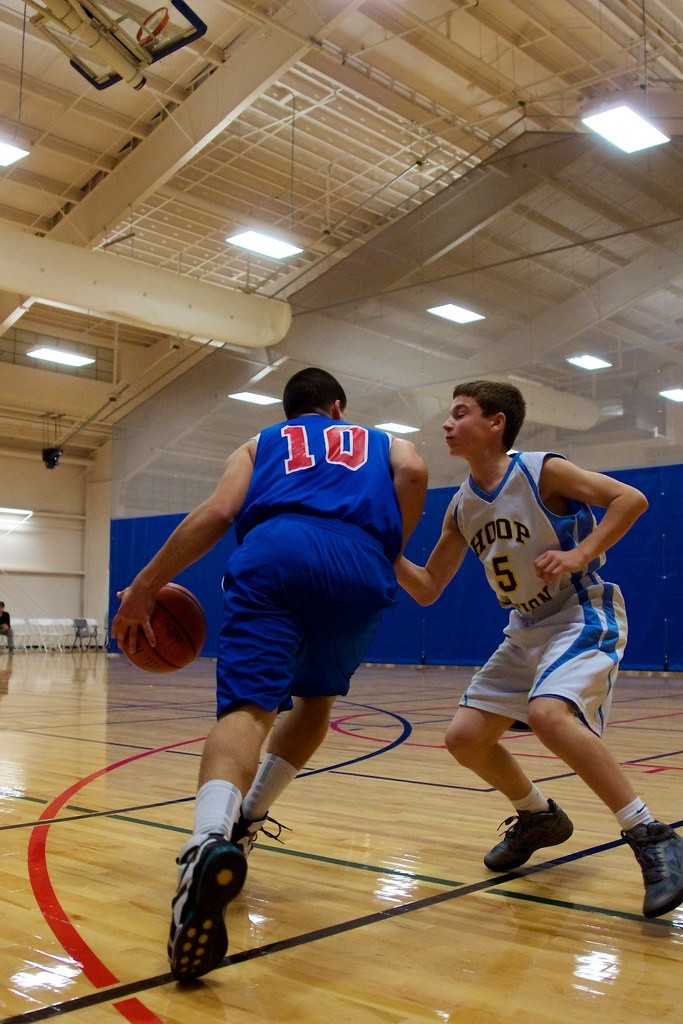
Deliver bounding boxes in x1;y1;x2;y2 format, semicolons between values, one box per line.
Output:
231;804;294;857
167;832;248;981
483;798;575;871
621;819;683;919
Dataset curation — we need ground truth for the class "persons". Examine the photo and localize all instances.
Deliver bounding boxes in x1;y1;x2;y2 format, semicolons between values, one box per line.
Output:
0;601;16;655
112;367;429;981
395;382;682;921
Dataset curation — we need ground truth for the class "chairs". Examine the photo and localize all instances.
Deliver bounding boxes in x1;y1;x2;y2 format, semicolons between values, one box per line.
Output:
0;617;102;655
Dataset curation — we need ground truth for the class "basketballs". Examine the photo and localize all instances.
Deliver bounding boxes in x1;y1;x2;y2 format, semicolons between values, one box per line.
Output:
119;580;204;674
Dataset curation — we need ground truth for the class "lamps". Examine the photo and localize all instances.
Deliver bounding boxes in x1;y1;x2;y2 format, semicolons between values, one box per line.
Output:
566;254;614;370
25;308;97;367
0;3;30;168
225;93;304;260
581;0;672;155
426;236;488;324
